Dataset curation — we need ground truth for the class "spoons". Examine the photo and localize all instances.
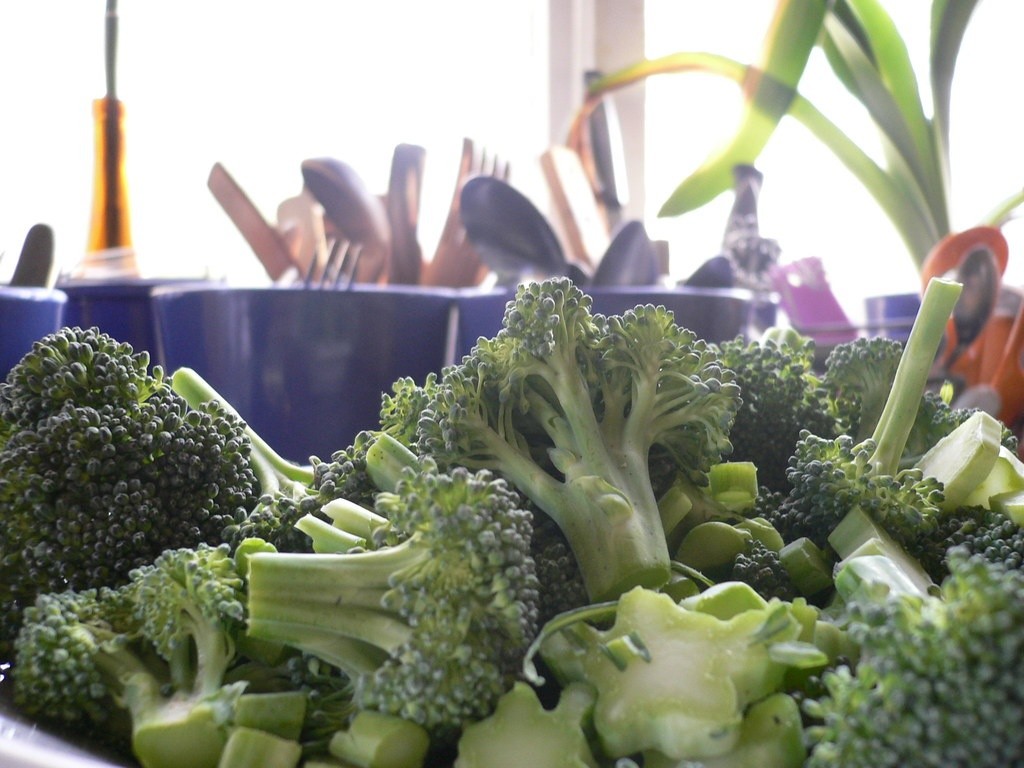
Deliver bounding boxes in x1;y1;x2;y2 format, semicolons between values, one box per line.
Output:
940;243;1001;372
592;222;657;286
459;178;568;286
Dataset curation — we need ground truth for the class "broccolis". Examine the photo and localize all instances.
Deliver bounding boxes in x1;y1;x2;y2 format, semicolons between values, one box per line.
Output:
0;281;1024;768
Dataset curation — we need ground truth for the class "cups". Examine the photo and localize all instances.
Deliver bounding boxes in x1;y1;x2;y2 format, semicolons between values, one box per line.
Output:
56;277;222;366
0;285;67;383
458;275;779;422
156;286;458;462
859;291;948;370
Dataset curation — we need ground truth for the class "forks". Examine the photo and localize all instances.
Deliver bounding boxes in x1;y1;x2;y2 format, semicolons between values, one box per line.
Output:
427;139;511;286
305;239;364;291
